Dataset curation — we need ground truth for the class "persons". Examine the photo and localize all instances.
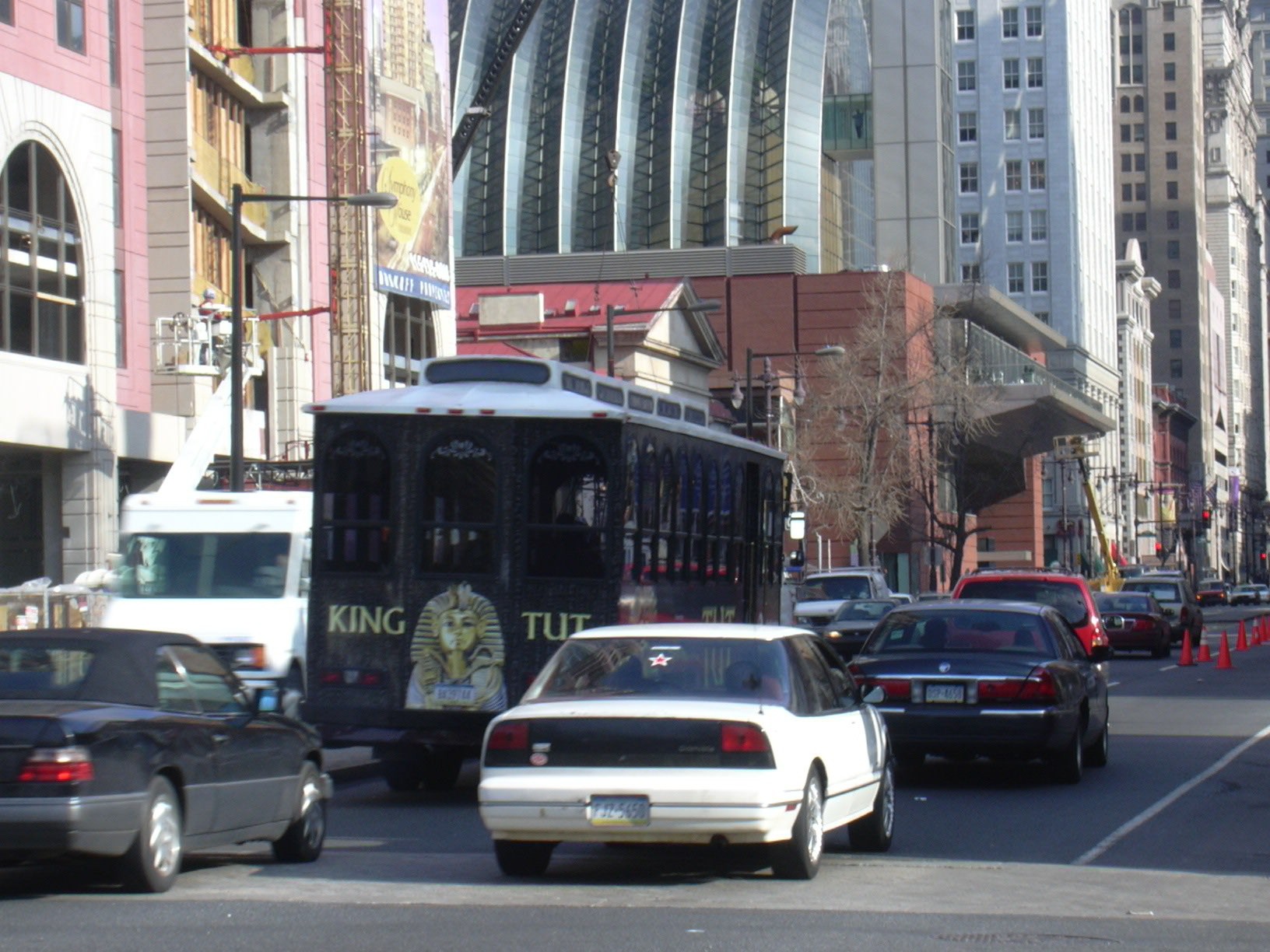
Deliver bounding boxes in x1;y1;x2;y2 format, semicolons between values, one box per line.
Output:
405;583;507;711
199;288;225;365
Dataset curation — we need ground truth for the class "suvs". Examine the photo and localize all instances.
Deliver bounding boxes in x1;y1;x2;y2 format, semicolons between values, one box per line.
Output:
1117;570;1205;649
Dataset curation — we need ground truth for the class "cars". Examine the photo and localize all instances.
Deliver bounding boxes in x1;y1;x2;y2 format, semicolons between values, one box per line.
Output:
1089;589;1176;661
1195;578;1228;604
934;566;1110;661
792;563;956;664
477;621;897;881
1231;582;1270;607
0;624;335;895
846;596;1111;785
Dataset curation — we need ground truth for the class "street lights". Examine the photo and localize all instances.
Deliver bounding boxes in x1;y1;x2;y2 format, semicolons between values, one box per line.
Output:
745;343;846;441
229;181;402;492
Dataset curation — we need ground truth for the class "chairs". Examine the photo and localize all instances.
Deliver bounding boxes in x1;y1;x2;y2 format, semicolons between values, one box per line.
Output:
0;670;52;690
758;677;783;700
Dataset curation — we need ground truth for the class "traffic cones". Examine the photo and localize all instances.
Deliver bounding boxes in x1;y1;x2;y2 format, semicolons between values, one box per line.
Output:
1249;617;1262;647
1195;625;1213;662
1233;619;1250;651
1259;615;1270;642
1214;630;1233;669
1175;628;1198;667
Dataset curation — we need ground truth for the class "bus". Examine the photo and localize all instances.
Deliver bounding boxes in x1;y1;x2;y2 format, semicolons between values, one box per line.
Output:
293;353;809;794
86;489;316;718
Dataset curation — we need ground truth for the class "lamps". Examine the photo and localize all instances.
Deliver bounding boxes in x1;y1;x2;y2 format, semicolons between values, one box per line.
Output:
466;105;493;117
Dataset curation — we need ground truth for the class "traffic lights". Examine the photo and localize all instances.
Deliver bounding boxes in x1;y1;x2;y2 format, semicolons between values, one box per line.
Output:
1202;509;1212;529
